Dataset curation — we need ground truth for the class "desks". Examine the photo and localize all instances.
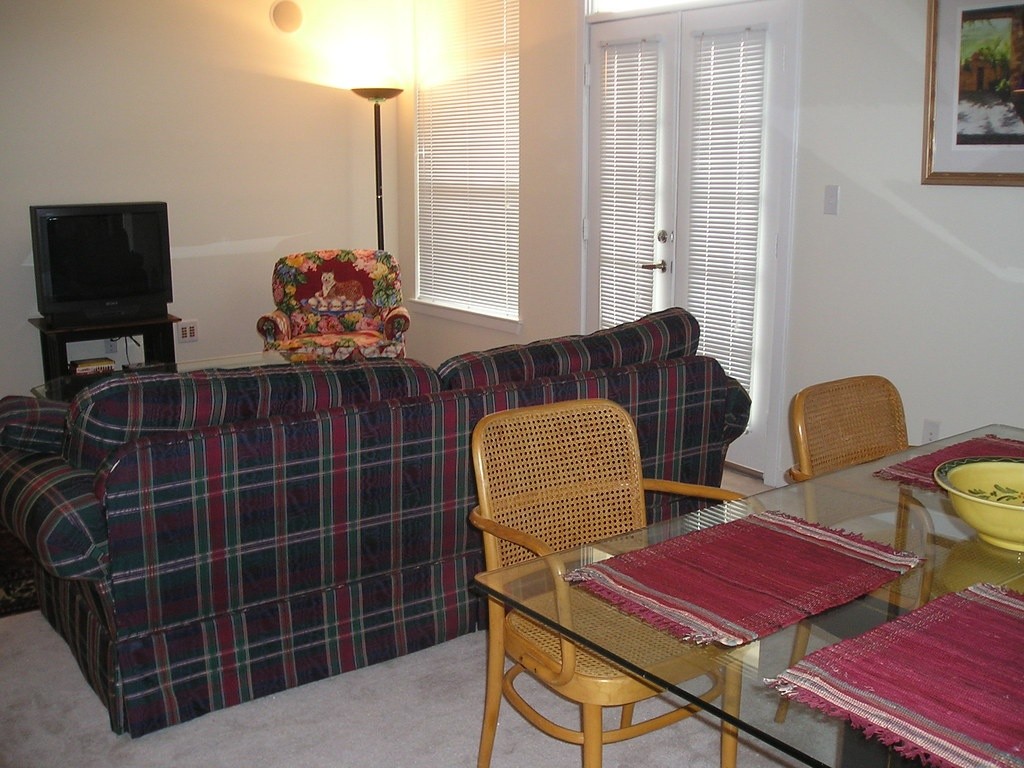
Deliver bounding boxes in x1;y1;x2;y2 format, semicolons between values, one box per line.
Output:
473;424;1024;768
28;313;181;407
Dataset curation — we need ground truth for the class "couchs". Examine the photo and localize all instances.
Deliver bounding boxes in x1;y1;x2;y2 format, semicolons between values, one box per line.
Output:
0;305;752;741
256;251;411;364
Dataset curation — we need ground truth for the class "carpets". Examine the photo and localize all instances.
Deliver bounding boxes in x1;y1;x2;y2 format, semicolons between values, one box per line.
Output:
0;518;40;619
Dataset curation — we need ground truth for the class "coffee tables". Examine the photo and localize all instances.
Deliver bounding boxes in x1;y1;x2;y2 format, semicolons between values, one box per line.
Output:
30;350;292;401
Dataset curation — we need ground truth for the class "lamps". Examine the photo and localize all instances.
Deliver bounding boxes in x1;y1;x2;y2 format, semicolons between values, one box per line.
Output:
351;88;403;250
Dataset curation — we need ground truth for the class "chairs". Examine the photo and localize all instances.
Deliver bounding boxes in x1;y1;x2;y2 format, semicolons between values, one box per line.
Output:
470;399;742;768
772;376;960;726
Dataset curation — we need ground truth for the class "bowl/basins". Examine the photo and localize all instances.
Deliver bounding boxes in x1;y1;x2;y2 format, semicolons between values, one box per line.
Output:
935;455;1024;552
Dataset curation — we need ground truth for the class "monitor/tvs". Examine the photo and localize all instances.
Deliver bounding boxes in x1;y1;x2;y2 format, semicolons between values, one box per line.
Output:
29;201;173;329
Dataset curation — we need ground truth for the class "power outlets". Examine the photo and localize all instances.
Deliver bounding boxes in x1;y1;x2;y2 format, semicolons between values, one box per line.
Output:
920;418;940;442
104;338;118;354
178;319;198;343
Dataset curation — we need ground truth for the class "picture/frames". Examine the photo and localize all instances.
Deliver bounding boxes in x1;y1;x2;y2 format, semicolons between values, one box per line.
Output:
920;1;1024;188
